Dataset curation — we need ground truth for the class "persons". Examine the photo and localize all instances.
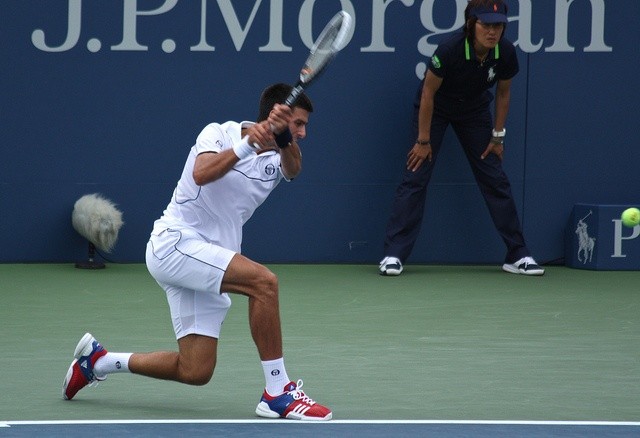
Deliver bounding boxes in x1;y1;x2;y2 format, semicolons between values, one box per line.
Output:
378;0;545;275
63;83;334;422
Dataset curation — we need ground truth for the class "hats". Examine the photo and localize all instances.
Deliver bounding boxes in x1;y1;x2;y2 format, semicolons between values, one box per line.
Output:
466;2;509;23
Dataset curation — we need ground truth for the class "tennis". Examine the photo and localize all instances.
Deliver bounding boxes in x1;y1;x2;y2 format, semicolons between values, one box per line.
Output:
622;207;640;227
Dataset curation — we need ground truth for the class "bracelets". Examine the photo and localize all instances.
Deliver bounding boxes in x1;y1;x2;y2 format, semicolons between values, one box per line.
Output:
231;134;260;160
272;125;293;149
490;138;504;145
416;139;432;145
492;128;506;138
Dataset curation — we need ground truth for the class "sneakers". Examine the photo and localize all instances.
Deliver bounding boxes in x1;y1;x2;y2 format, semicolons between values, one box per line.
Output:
502;256;544;275
61;332;108;400
380;256;404;275
255;379;333;421
